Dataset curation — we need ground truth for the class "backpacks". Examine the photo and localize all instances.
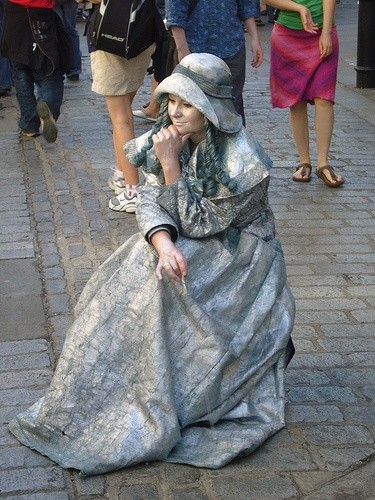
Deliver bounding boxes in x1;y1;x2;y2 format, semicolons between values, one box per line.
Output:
148;28;180;82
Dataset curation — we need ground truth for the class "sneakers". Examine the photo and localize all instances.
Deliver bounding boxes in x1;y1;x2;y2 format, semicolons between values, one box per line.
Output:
108;175;127;195
108;190;138;213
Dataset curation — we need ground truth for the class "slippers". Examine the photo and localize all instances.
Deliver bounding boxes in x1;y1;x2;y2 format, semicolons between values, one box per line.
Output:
132;110;158;122
315;163;345;188
255;20;265;26
292;162;312;182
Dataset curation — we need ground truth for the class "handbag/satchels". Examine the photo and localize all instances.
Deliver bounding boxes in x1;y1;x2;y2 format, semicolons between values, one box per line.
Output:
89;0;167;60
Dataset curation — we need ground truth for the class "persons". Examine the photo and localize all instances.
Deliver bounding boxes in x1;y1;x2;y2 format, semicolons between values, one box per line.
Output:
8;53;296;478
262;0;346;186
132;0;262;127
83;0;167;213
0;0;82;142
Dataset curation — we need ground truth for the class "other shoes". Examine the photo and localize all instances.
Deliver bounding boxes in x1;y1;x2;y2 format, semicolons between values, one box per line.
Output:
0;89;8;95
36;101;59;144
67;74;80;81
20;129;41;137
336;1;341;4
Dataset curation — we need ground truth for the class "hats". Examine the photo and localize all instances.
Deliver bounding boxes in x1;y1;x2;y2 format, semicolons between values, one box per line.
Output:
152;53;243;134
